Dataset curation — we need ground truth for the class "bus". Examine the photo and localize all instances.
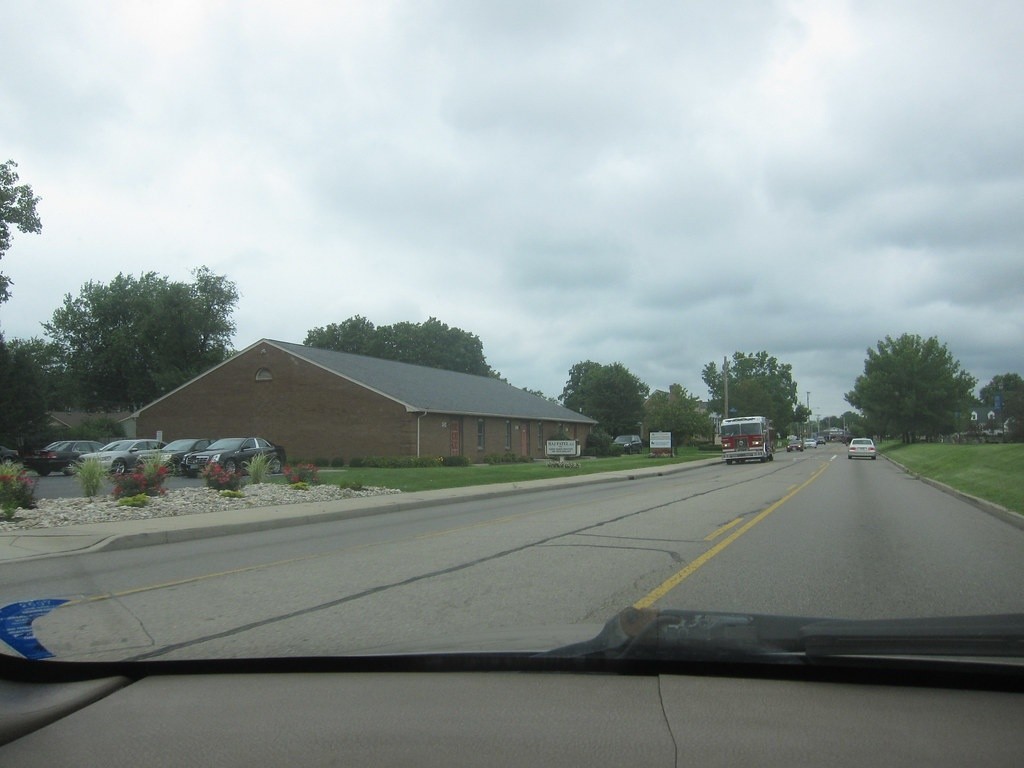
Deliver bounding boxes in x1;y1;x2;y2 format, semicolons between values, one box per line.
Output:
720;416;777;465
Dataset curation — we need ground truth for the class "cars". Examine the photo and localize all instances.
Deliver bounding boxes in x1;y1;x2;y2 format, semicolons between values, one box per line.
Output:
78;439;167;476
817;437;826;445
612;435;644;455
135;438;217;474
0;604;1023;766
0;445;17;465
181;437;287;478
803;439;816;449
787;440;803;452
24;441;106;477
847;438;877;459
